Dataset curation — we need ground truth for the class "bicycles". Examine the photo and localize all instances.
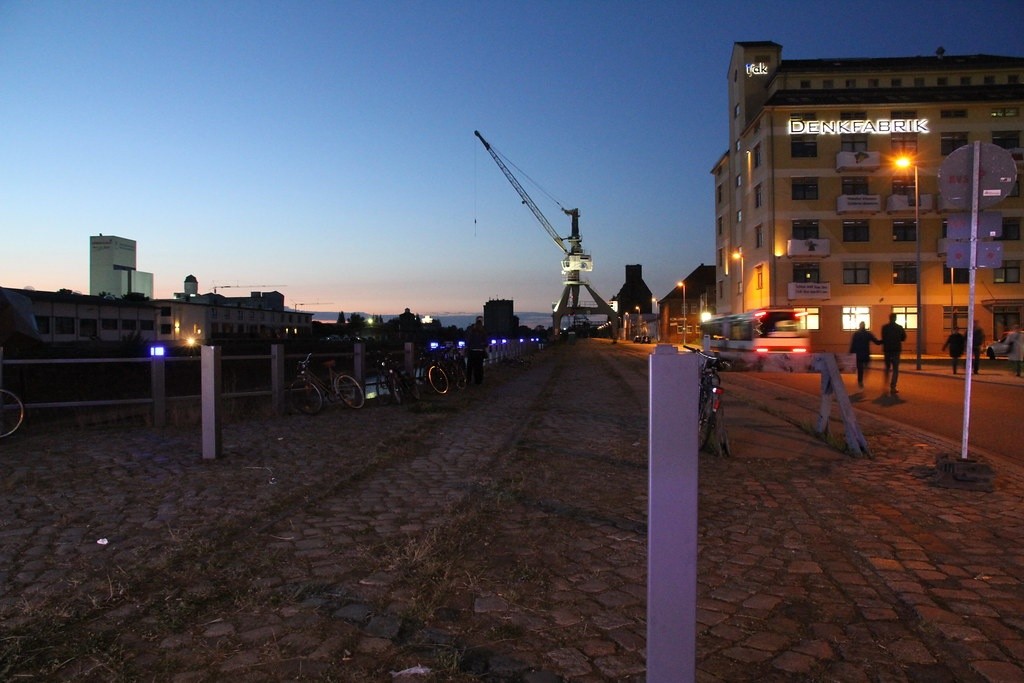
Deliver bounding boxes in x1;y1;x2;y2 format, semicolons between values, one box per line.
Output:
373;350;420;406
420;348;468;394
683;344;732;458
290;353;365;415
0;388;25;439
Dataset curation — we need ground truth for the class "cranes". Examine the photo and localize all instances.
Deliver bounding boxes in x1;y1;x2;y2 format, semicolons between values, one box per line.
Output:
474;130;620;342
213;285;290;298
293;302;334;314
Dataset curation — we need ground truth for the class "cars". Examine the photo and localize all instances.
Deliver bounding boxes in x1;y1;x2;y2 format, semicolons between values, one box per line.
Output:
633;336;641;343
987;331;1024;360
641;335;651;344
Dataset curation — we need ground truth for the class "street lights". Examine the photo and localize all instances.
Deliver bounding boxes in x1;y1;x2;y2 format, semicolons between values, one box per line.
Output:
734;253;744;314
636;307;640;317
651;298;658;343
895;156;923;371
679;283;686;345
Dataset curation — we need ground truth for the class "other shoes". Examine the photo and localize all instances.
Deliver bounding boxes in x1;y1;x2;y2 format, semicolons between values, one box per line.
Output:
890;387;899;393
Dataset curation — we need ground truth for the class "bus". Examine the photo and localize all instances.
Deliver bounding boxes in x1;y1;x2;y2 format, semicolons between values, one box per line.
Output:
700;308;810;372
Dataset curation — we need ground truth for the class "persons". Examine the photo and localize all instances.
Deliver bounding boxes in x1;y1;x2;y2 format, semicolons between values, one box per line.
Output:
942;320;983;374
1006;325;1024;376
463;316;489;387
850;313;907;393
